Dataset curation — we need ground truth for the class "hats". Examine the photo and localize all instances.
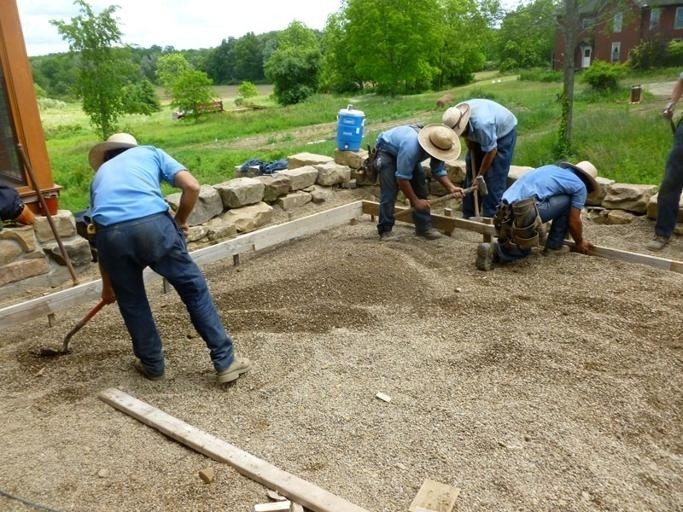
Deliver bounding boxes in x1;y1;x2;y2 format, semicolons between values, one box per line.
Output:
559;160;599;198
442;103;470;137
89;133;140;172
417;123;461;162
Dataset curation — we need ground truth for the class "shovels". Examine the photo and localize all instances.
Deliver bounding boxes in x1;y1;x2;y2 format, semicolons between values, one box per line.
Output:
33;301;106;356
468;149;491;224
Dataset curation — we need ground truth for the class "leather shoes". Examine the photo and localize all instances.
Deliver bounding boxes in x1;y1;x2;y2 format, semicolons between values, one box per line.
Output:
217;357;251;383
134;359;163;381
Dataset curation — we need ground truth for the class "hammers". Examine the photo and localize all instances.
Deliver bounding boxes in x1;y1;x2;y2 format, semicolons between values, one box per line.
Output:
393;176;489;220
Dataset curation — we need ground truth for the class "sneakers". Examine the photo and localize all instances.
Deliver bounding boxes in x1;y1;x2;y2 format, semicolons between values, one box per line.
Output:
543;245;570;256
379;231;400;242
476;243;495;271
647;235;673;252
416;227;442;240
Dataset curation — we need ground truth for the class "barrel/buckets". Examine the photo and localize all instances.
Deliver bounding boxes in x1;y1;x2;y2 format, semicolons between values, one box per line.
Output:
38;196;57;215
336;104;366;151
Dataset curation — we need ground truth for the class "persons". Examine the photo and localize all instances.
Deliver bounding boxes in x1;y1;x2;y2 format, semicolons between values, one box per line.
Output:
375;122;461;243
475;161;598;271
440;98;517;223
645;72;683;250
85;132;252;384
1;180;39;225
